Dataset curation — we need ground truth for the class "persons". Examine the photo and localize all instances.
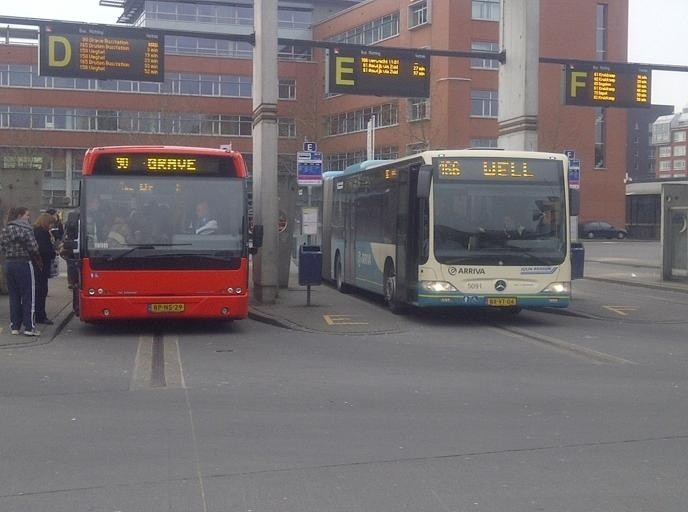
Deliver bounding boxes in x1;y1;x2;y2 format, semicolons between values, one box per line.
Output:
49;208;64;242
185;199;219;234
0;206;41;337
28;212;58;326
69;196;100;319
64;213;74;288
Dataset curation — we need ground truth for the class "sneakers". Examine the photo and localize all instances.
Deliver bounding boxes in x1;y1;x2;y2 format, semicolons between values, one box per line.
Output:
12;329;23;335
35;318;52;324
23;327;41;336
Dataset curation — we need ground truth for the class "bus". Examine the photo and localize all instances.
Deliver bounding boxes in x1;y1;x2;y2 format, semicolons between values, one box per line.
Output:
320;148;570;311
77;143;250;320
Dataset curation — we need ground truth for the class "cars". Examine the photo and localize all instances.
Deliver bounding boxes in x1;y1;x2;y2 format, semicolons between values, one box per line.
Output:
579;221;628;240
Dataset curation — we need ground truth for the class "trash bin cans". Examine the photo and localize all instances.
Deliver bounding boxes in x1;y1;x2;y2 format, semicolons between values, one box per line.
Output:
298;245;322;286
571;242;585;280
